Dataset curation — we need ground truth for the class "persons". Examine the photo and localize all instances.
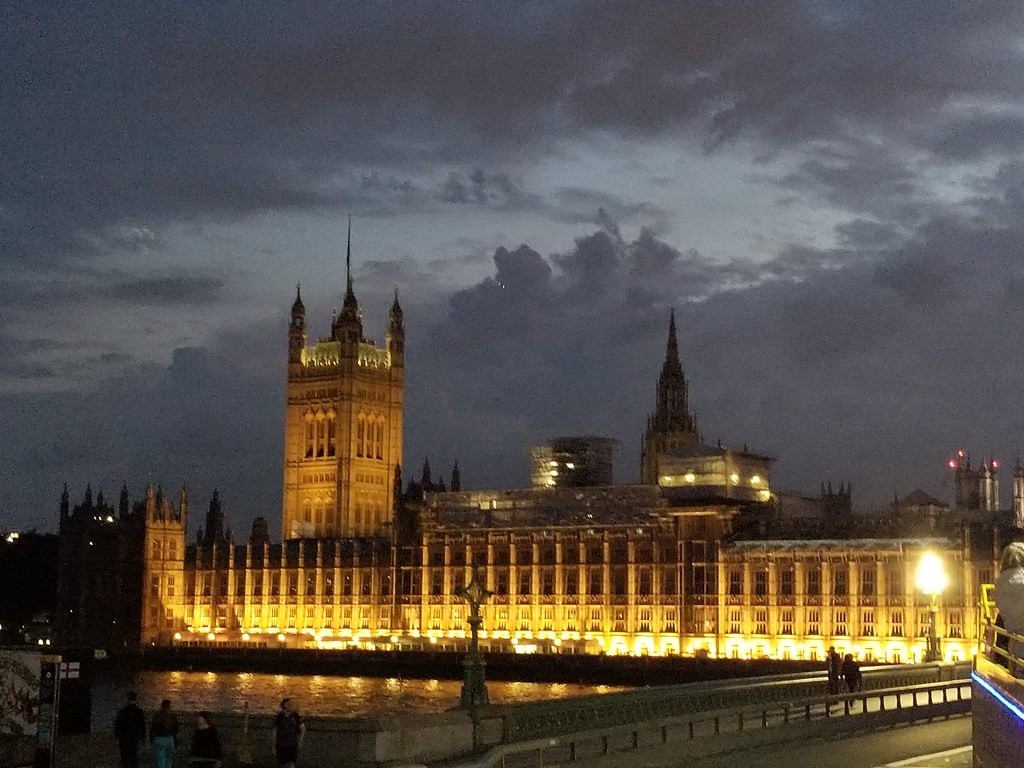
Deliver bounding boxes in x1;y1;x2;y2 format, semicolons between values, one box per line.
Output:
270;697;306;768
149;698;181;768
991;541;1023;679
113;690;146;768
826;646;863;709
186;710;223;768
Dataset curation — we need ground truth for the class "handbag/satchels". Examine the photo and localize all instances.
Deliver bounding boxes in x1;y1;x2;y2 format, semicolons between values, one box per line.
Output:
174;735;179;750
